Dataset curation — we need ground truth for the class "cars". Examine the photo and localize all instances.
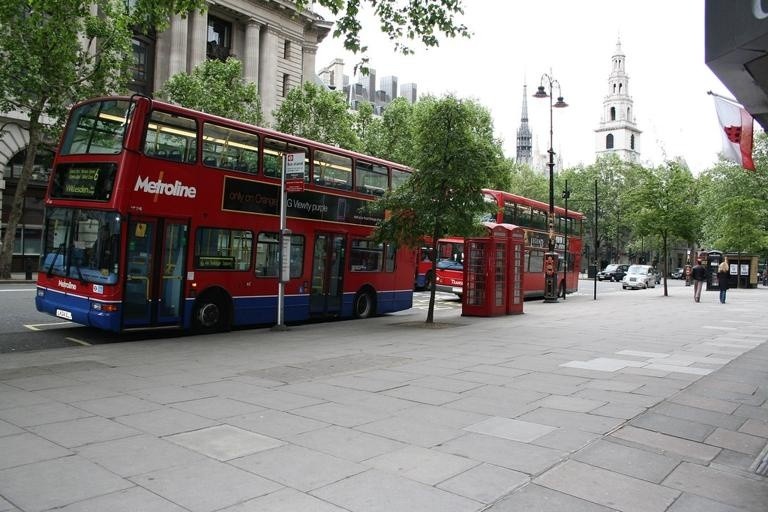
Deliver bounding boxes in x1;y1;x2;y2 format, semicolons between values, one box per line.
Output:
655;270;661;284
669;268;684;280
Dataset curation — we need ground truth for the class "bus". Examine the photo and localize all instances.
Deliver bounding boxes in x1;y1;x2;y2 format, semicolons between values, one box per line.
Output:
433;188;582;303
415;232;438;290
35;93;426;337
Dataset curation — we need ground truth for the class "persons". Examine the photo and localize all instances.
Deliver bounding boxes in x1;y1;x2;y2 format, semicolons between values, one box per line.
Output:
717;262;730;304
684;261;692;286
691;259;706;303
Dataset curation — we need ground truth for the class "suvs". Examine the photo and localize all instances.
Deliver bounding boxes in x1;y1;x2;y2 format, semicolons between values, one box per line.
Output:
621;265;655;290
597;264;628;281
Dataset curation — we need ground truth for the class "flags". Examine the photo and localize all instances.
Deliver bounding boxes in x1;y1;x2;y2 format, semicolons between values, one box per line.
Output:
713;90;757;173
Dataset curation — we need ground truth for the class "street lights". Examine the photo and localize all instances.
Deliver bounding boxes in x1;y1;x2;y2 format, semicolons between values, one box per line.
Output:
533;73;568;305
